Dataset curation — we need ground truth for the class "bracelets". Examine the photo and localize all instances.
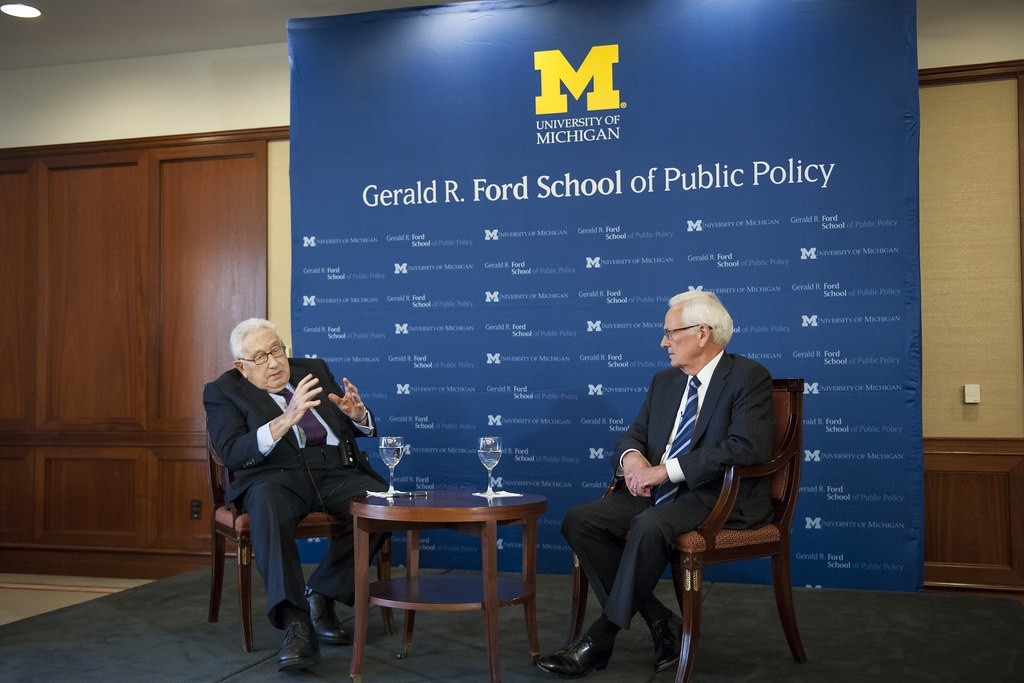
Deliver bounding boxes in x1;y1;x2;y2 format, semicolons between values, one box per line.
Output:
352;406;369;422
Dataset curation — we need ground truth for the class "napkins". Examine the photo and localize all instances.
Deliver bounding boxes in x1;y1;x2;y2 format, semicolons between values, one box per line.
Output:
471;491;524;500
365;490;407;498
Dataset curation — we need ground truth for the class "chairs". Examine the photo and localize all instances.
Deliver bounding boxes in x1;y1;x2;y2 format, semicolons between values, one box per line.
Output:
206;414;396;654
567;378;809;683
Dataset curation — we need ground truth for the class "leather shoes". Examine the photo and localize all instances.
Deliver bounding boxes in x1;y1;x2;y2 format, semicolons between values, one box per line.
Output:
537;633;614;680
276;619;321;672
648;612;684;673
303;586;347;644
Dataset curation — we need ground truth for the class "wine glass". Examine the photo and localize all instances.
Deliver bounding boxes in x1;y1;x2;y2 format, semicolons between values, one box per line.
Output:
379;437;405;498
477;436;503;496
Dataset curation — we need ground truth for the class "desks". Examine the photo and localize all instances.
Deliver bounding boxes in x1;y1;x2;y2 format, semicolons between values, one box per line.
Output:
349;490;548;683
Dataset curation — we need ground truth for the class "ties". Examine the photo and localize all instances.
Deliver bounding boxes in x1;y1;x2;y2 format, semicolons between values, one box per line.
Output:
274;388;328;448
655;376;702;507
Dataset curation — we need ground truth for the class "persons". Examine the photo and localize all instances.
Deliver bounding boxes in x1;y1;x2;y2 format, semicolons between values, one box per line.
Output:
535;291;776;680
202;318;389;672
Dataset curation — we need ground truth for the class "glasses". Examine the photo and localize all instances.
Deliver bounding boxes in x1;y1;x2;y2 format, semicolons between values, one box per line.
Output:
663;324;713;340
238;340;286;365
